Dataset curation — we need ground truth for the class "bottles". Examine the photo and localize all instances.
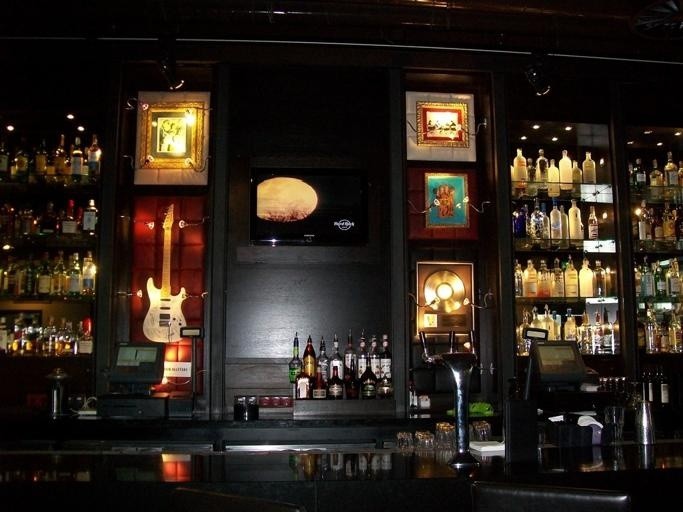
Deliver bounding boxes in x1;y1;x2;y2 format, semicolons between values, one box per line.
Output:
287;452;393;481
512;145;616;355
0;248;97;298
1;131;104;186
640;364;671;407
0;311;95;355
632;148;683;355
0;196;98;240
289;328;393;398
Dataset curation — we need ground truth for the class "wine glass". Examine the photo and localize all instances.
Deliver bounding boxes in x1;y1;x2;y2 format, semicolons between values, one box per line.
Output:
629;381;641;404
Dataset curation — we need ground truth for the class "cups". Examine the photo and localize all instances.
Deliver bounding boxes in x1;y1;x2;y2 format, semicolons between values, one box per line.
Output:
597;374;631;393
261;392;292;410
394;420;491;451
630;398;656;445
636;448;657;470
604;407;626;442
397;451;458;465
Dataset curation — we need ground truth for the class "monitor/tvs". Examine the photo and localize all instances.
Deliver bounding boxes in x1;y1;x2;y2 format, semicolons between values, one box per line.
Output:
248;167;369;246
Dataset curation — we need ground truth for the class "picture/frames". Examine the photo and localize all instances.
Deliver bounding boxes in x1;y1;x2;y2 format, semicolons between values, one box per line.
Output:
134;91;211;187
408;168;480;240
403;90;479;166
415;261;474;344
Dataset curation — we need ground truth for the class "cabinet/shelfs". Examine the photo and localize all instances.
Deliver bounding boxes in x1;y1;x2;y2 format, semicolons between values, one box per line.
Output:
0;132;105;396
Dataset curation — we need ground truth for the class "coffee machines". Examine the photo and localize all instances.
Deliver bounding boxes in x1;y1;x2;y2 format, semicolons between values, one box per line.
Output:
47;369;76;420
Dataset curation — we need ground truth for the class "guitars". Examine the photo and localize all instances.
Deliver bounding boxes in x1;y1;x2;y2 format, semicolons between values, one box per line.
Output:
144;203;187;343
